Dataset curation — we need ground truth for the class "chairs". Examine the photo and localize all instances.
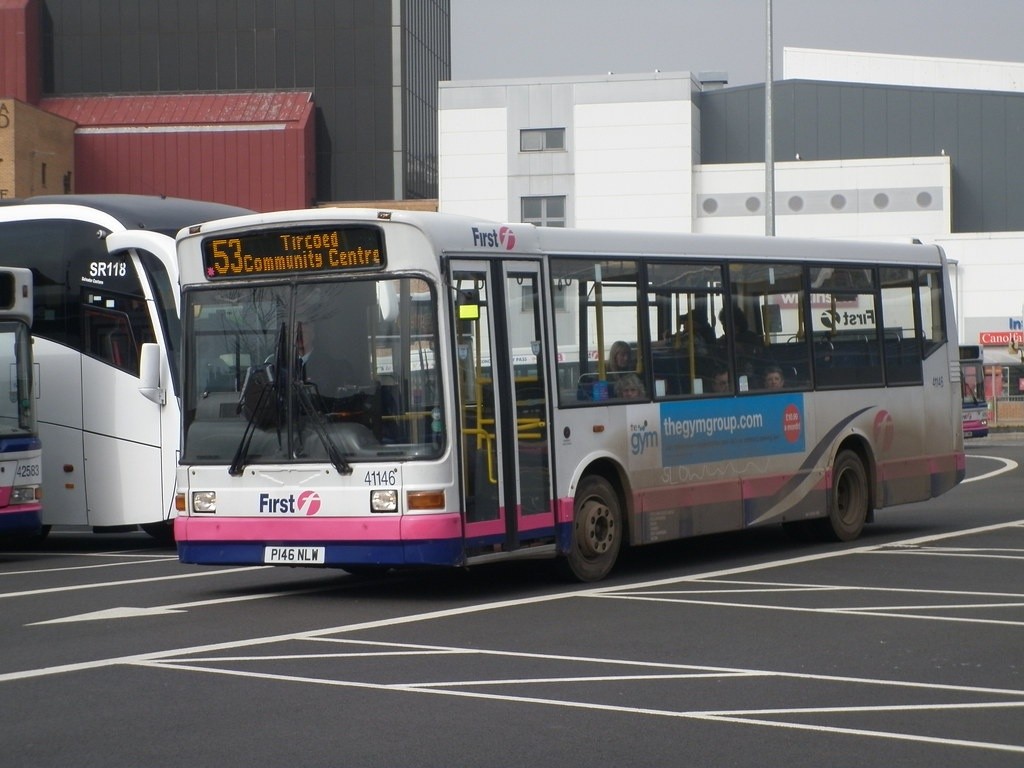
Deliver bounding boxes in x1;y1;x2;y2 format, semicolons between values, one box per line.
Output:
576;327;926;400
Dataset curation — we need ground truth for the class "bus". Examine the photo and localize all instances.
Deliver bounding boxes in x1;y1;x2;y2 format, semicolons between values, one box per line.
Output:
0;266;45;551
170;204;968;588
0;197;264;539
959;344;989;439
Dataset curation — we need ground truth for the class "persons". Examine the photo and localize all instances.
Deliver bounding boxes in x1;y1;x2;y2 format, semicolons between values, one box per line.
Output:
663;306;784;393
282;316;341;412
596;341;631;381
